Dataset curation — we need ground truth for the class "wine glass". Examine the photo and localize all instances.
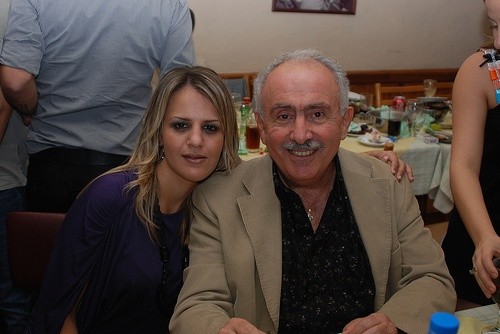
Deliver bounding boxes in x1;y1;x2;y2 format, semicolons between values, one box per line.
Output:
481;257;500;334
407;99;424;137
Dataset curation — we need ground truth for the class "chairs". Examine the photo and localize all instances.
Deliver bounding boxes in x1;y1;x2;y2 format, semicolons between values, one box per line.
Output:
5;212;77;334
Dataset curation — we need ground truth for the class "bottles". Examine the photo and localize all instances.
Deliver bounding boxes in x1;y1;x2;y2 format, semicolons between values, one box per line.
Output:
429;311;459;334
239;97;250;154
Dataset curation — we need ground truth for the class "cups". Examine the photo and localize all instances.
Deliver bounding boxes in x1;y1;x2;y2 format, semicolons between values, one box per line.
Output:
423;79;437;97
360;92;373;114
246;115;260;153
388;106;403;136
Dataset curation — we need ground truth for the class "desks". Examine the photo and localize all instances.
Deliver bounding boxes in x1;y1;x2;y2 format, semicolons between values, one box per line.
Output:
238;125;455;215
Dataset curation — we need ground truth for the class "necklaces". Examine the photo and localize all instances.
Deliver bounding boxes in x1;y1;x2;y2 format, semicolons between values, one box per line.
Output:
307;189;326;220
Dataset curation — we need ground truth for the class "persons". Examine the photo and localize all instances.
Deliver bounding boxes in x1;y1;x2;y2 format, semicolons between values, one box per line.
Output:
441;0;500;311
32;66;415;334
0;0;28;334
170;49;457;334
0;0;196;213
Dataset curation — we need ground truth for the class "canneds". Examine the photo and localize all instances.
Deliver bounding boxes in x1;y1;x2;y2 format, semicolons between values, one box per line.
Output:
392;96;407;112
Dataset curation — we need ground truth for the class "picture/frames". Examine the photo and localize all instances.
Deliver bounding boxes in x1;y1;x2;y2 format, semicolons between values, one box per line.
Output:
272;0;357;15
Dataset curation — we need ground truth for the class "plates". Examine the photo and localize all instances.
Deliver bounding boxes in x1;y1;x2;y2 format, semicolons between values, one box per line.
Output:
358;137;391;147
347;126;371;136
417;97;448;102
